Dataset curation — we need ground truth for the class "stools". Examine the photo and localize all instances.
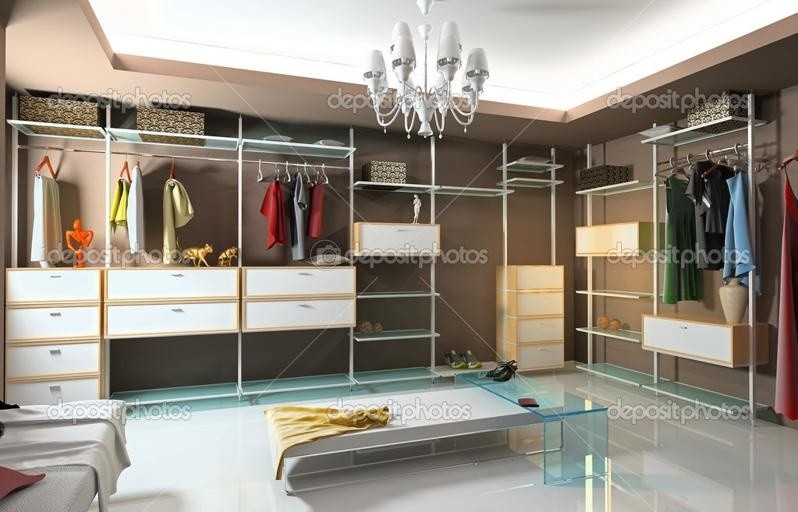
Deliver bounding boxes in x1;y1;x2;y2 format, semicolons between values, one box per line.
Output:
0;400;128;512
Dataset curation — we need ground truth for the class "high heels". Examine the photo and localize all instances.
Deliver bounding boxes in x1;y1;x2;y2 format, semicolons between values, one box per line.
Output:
486;360;518;382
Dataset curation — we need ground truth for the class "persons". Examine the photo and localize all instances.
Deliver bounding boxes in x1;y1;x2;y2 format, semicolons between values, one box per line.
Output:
413;194;422;224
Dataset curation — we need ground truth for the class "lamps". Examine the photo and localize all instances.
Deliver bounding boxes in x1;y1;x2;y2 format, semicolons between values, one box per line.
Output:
358;0;493;141
259;372;610;494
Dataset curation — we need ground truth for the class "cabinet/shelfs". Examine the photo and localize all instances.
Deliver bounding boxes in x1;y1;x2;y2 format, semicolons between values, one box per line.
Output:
347;131;564;384
5;95;360;405
571;94;770;427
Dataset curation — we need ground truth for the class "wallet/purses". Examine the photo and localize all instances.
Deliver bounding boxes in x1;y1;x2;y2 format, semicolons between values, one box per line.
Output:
518;398;539;407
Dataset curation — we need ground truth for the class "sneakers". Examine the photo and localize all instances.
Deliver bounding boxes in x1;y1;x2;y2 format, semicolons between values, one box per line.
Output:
443;350;482;369
